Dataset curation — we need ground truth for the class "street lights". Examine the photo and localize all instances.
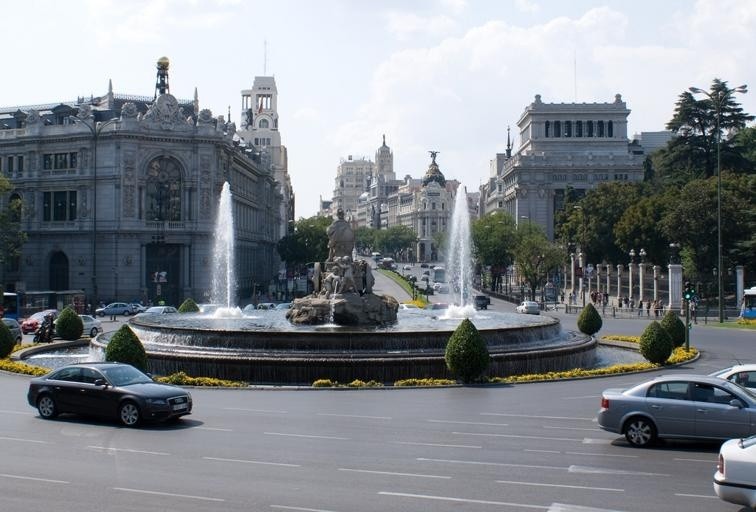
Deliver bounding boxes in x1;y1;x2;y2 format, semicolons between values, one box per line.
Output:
638;248;647;263
484;225;490;240
497;220;506;241
668;241;682;265
519;215;532;239
68;113;124;313
683;82;750;324
627;249;636;264
573;204;587;252
712;265;735;321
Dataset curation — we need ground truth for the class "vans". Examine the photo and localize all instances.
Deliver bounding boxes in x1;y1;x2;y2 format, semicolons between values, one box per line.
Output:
470;294;490;310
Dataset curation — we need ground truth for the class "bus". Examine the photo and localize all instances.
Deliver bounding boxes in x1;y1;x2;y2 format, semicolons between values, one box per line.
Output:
743;285;756;320
2;288;87;320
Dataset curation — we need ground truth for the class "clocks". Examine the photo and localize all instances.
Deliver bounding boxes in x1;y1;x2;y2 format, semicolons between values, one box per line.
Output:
156;93;178;116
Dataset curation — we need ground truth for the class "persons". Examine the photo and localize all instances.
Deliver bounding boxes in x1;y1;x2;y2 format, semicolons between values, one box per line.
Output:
646;300;651;316
568;292;576;304
638;301;643;315
41;313;54;343
653;300;663;316
618;295;634;312
591;290;608;307
319;208;360;299
561;291;564;301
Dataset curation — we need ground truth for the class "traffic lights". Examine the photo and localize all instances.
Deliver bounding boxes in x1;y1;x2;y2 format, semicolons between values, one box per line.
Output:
690;284;696;294
696;282;703;293
684;281;691;301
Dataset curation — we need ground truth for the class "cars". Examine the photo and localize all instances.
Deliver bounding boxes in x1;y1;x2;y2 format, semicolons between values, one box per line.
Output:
371;251;452;295
700;363;756;397
0;301;179;347
711;433;756;511
515;300;541;315
195;302;292;315
596;359;756;448
25;360;194;429
